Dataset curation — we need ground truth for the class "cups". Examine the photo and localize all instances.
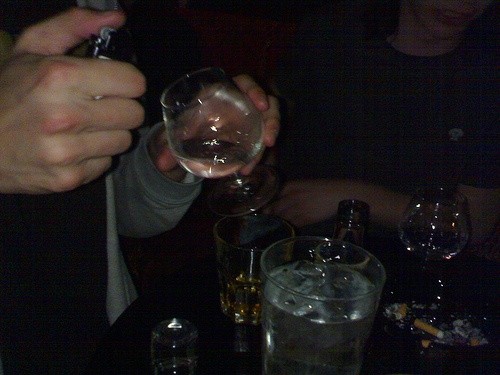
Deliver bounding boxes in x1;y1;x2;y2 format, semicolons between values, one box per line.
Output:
260;235;386;375
214;214;296;324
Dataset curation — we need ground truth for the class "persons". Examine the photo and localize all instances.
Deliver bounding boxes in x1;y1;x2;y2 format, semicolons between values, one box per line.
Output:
0;0;280;375
234;0;500;263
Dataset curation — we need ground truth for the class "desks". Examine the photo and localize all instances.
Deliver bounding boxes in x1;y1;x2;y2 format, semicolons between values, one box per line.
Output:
83;217;500;375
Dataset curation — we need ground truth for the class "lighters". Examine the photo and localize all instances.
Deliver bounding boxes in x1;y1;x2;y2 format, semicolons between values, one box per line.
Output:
85;27;130;100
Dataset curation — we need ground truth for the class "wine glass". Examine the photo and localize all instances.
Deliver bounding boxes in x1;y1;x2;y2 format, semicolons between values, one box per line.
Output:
160;65;279;216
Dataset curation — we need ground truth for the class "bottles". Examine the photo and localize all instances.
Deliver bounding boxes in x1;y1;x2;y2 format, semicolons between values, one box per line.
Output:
331;199;371;248
149;321;197;375
398;166;470;302
